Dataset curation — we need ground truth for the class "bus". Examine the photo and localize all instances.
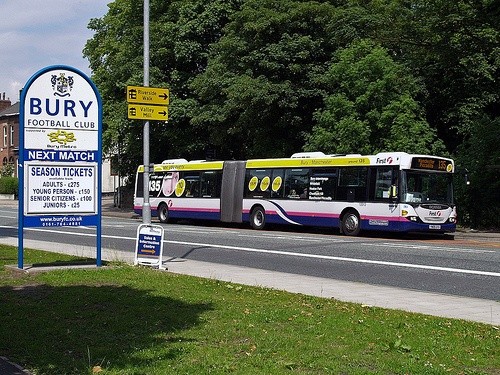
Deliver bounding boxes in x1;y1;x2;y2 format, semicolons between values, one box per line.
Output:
133;151;471;237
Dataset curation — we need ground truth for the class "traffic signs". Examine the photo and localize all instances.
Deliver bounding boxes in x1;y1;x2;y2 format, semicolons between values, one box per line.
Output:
127;103;169;121
126;86;169;105
